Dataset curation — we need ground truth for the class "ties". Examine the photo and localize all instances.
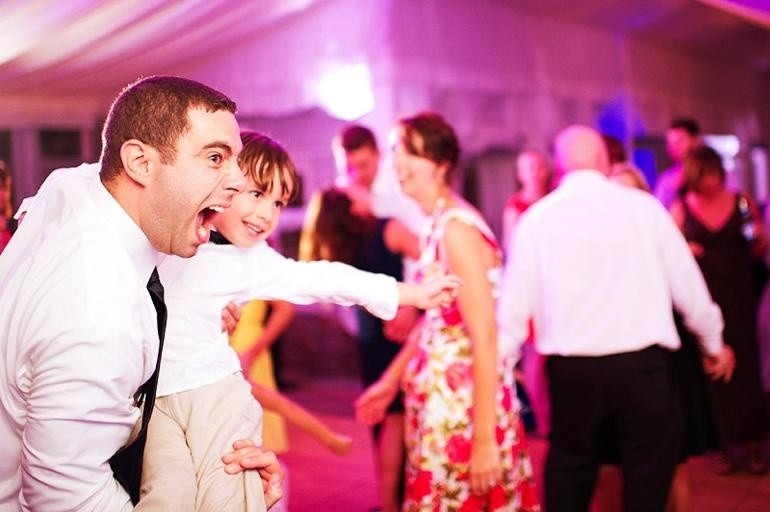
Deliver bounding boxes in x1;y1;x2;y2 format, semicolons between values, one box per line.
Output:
107;263;168;508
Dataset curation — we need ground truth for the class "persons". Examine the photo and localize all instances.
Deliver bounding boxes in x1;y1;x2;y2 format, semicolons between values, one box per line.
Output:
0;74;770;512
